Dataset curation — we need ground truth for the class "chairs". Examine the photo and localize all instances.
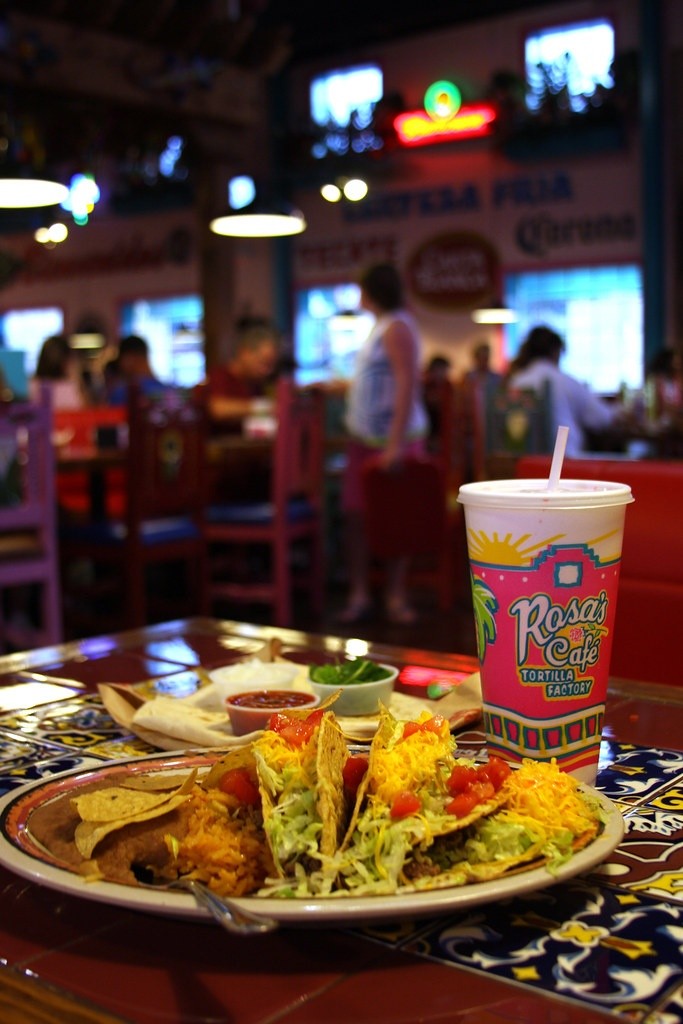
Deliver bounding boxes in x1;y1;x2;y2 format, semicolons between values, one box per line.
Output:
0;377;484;653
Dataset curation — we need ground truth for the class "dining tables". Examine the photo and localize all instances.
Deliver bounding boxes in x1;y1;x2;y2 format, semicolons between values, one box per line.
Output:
0;617;683;1024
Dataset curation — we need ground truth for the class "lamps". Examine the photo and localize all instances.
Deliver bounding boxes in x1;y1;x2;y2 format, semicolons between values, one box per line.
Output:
0;149;70;208
206;186;307;236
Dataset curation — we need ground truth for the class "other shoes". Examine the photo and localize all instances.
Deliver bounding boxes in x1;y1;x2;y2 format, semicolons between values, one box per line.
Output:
388;603;421;625
336;596;373;623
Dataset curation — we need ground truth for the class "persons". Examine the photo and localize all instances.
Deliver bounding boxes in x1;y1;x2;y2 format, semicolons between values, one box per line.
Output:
0;316;281;645
628;351;683;458
424;356;453;433
343;261;431;622
496;327;632;455
462;345;503;413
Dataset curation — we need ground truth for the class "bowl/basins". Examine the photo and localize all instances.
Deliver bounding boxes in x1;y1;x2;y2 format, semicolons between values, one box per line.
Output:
308;661;399;717
225;689;320;736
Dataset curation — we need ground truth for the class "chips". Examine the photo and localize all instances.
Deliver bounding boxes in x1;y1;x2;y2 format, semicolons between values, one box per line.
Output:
70;686;343;862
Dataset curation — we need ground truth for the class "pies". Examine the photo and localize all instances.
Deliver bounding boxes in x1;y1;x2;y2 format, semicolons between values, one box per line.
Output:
250;699;601;894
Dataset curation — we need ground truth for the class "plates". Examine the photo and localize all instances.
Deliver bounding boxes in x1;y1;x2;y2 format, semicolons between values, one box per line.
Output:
0;744;624;920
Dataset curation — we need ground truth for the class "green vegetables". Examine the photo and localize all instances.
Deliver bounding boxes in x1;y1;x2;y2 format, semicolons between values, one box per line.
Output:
308;658;393;685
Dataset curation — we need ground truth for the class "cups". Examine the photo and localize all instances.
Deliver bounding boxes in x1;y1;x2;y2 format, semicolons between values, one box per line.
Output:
455;478;635;790
